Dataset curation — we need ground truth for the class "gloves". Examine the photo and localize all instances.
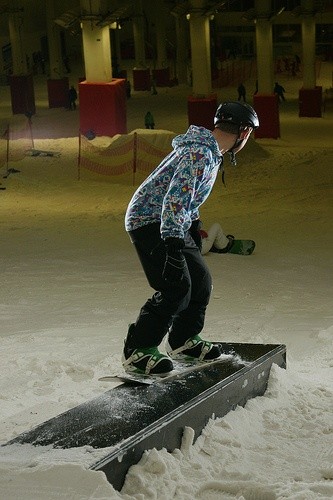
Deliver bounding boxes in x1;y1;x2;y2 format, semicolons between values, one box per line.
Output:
191;220;203;252
162;238;191;290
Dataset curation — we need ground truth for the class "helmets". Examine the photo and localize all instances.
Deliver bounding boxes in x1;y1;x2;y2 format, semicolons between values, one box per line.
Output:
215;101;260;130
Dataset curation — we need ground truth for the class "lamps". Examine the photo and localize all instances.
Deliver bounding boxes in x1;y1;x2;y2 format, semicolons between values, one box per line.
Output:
184;9;193;21
209;7;217;21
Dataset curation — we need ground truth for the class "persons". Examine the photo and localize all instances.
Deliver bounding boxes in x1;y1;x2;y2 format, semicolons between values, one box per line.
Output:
283;55;300;76
273;82;285;102
144;112;155;129
237;83;247;102
64;85;77;110
121;101;260;379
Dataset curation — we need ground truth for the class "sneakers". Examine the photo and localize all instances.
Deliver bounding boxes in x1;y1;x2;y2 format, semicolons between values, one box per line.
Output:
120;347;174;377
165;335;222;362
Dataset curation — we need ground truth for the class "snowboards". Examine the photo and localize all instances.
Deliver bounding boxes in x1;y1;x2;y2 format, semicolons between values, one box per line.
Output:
98;349;232;390
209;239;256;256
